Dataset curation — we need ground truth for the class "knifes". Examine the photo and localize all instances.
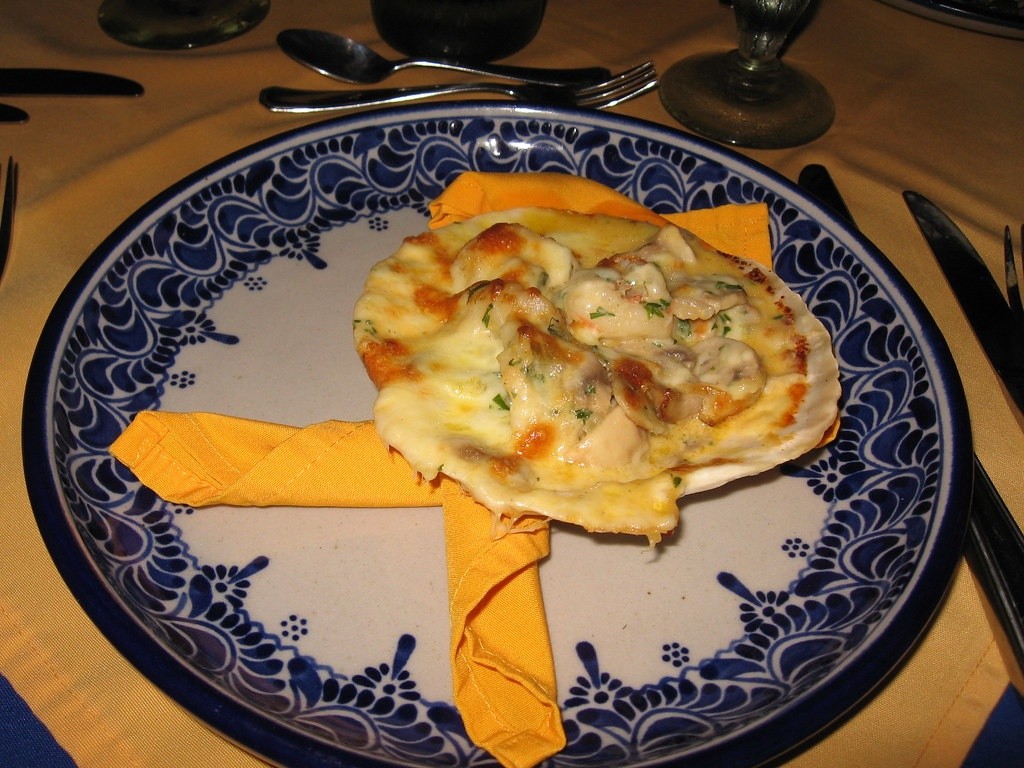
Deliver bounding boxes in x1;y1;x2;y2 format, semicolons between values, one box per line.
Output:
796;163;1024;671
0;68;144;98
902;188;1024;415
0;103;30;123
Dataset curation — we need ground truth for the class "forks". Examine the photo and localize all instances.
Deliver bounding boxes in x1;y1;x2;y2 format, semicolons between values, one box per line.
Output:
258;61;657;111
0;155;15;285
1003;224;1024;319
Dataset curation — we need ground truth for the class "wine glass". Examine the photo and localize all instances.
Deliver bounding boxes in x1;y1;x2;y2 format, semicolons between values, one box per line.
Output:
657;0;835;150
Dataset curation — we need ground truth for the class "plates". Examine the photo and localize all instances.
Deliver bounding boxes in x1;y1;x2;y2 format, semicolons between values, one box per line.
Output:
22;101;973;768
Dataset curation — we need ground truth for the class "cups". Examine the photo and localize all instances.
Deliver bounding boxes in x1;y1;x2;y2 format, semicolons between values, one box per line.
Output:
370;1;548;64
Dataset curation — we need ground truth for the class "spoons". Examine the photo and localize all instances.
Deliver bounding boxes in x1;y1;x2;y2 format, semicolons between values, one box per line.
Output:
276;27;612;90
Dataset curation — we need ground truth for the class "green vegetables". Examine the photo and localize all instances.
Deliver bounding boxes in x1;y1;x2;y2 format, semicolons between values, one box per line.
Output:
460;261;748;426
